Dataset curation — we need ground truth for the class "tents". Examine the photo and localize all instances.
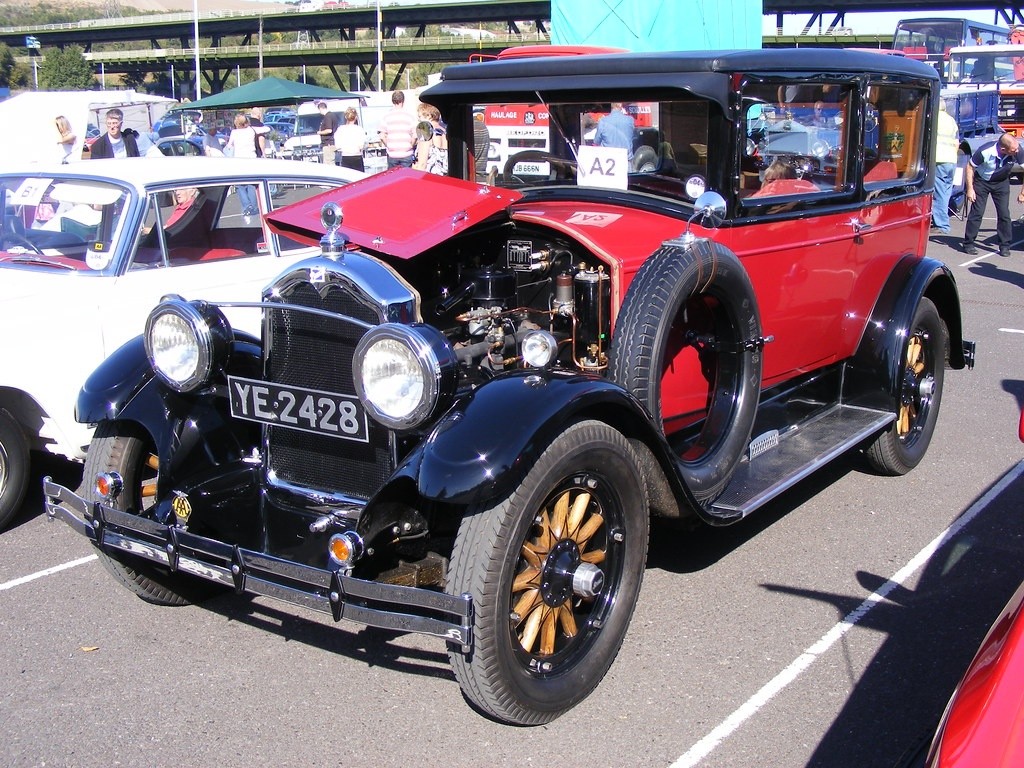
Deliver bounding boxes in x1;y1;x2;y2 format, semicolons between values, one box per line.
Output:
0;91;178;166
170;77;370;160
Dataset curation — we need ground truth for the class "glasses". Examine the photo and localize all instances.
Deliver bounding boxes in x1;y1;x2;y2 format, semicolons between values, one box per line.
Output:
106;121;121;126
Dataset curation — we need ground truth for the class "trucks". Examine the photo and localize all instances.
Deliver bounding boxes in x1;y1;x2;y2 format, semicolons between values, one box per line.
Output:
282;90;430;163
938;88;999;139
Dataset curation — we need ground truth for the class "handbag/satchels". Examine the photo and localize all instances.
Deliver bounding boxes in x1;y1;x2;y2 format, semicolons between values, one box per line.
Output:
252;126;262;157
223;143;235;156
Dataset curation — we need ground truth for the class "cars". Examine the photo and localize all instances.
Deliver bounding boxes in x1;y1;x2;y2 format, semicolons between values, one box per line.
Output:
0;158;371;532
147;107;297;156
41;40;978;728
895;410;1024;768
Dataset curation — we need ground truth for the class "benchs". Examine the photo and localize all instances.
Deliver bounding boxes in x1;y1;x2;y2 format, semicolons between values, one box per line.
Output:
132;246;246;264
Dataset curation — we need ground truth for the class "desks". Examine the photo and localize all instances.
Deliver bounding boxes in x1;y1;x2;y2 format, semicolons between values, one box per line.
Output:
276;150;342;168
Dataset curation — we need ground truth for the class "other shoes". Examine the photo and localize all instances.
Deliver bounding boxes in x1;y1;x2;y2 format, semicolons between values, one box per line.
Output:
931;227;947;234
1000;247;1010;257
963;245;978;255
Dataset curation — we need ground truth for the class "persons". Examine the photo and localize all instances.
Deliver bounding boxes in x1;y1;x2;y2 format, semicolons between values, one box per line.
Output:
335;108;365;171
378;91;417;167
759;162;796;188
124;128;158;156
227;114;270;215
593;103;634;173
962;133;1024;257
142;187;197;235
412;104;447;174
474;120;490;181
90;109;140;158
932;98;958;234
317;103;338;166
203;129;223;152
55;116;75;160
803;101;826;128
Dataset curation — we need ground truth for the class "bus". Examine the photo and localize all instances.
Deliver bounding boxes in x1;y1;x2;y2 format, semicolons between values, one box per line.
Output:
468;44;660;186
893;19;1013;140
946;44;1024;140
